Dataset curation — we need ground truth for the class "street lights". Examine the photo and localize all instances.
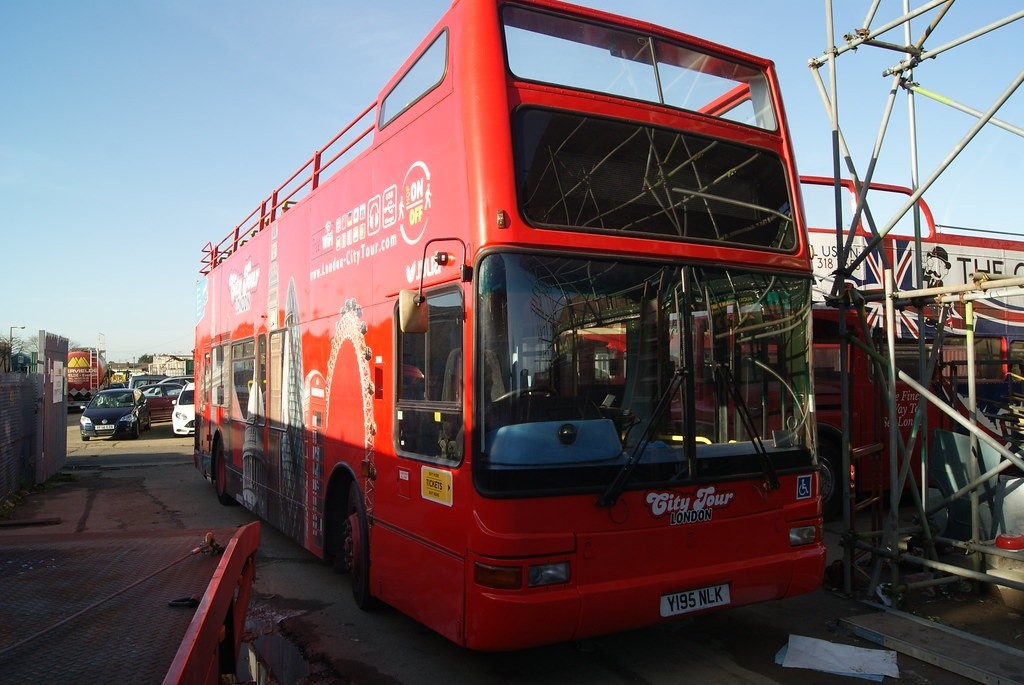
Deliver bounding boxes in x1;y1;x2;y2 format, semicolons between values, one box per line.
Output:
9;327;25;351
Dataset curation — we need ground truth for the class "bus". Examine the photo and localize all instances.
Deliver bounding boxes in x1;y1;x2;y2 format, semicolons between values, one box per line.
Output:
193;1;827;649
551;176;942;538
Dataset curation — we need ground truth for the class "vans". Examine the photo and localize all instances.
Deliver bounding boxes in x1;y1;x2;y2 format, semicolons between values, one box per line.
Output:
129;376;166;389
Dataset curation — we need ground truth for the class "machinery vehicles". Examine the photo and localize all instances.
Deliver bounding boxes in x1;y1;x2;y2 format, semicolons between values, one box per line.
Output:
61;347;113;412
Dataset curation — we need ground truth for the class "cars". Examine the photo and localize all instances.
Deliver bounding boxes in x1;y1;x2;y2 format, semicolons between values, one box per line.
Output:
79;387;152;441
109;381;129;390
136;383;187;424
144;376;194;399
172;382;201;436
